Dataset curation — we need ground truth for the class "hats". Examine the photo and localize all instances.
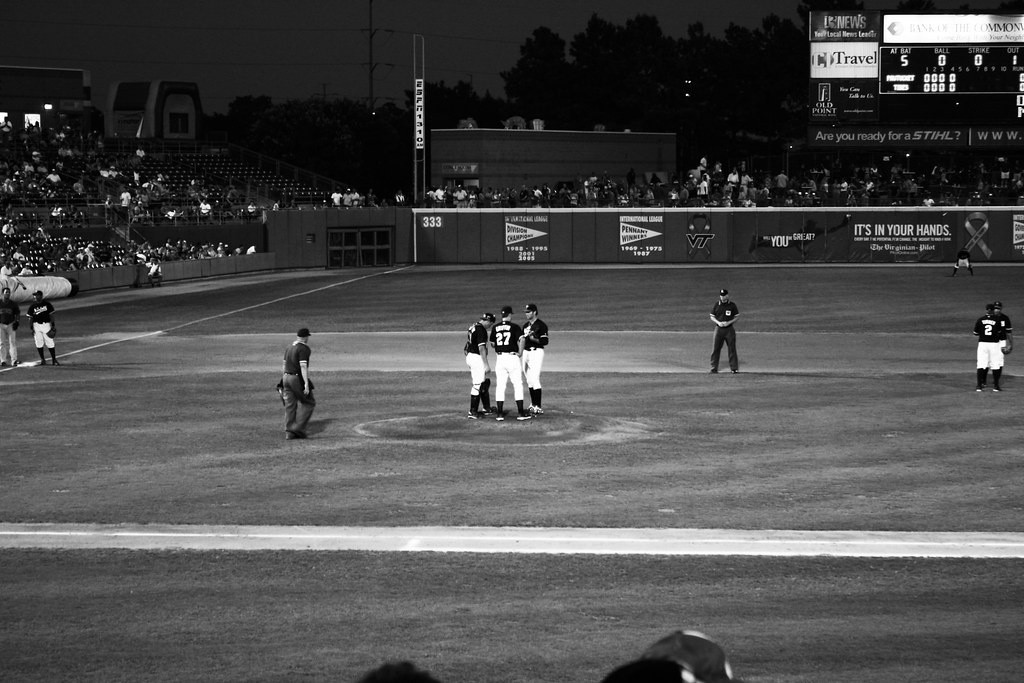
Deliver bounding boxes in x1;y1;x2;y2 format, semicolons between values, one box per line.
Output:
523;304;536;313
501;306;512;318
985;303;994;310
32;290;43;296
719;289;728;296
297;328;311;337
480;313;496;323
994;301;1002;309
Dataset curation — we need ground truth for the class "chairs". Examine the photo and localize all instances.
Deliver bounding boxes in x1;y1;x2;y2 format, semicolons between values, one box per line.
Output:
1;146;340;271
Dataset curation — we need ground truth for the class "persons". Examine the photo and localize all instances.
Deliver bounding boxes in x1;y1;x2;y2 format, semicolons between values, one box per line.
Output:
953;247;974;277
423;160;1024;208
1;289;61;367
358;657;700;682
971;302;1013;391
708;288;739;374
284;329;315;439
463;303;547;420
0;114;406;287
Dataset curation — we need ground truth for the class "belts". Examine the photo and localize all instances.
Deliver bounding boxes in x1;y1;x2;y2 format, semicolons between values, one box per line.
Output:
284;372;298;375
498;351;516;355
526;347;537;351
38;322;46;324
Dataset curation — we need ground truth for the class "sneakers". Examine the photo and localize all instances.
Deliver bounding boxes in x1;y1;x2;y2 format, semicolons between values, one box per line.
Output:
468;405;542;421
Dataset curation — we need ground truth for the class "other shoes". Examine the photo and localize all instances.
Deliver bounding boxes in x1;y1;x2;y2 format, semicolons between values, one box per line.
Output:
0;360;19;366
52;359;60;366
732;370;739;374
286;428;307;440
37;361;47;365
992;386;1002;392
711;367;718;373
976;385;987;392
